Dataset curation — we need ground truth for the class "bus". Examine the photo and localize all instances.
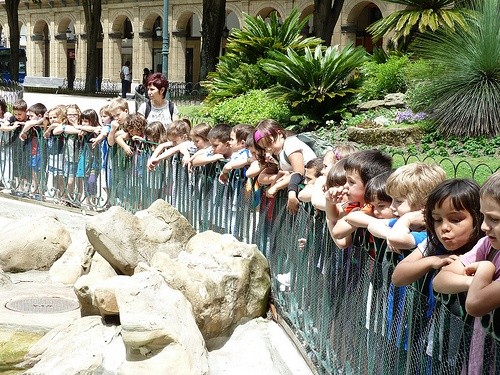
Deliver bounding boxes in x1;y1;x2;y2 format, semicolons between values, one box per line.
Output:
0;45;28;87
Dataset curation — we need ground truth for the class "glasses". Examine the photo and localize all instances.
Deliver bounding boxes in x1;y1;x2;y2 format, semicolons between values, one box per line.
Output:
67;114;79;117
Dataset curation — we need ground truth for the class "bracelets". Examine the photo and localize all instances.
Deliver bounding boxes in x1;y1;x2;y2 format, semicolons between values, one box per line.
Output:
61;125;65;130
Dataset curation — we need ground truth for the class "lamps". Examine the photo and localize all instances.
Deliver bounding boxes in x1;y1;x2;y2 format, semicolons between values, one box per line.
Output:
65;28;78;49
155;26;169;39
1;32;7;43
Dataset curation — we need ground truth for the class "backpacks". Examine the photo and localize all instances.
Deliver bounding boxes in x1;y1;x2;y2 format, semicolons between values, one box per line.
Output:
120;67;126;80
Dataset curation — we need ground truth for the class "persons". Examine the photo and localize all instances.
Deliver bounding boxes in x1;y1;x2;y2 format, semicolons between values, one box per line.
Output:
142;68;153;97
0;95;130;212
115;113;317;258
137;72;180;128
121;60;132;98
277;142;499;375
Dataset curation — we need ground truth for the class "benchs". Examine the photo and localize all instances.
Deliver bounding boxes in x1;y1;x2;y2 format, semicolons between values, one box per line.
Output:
22;76;65;95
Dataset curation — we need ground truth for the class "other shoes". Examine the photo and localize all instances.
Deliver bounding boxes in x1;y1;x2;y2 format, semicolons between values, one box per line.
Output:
66;197;80;208
